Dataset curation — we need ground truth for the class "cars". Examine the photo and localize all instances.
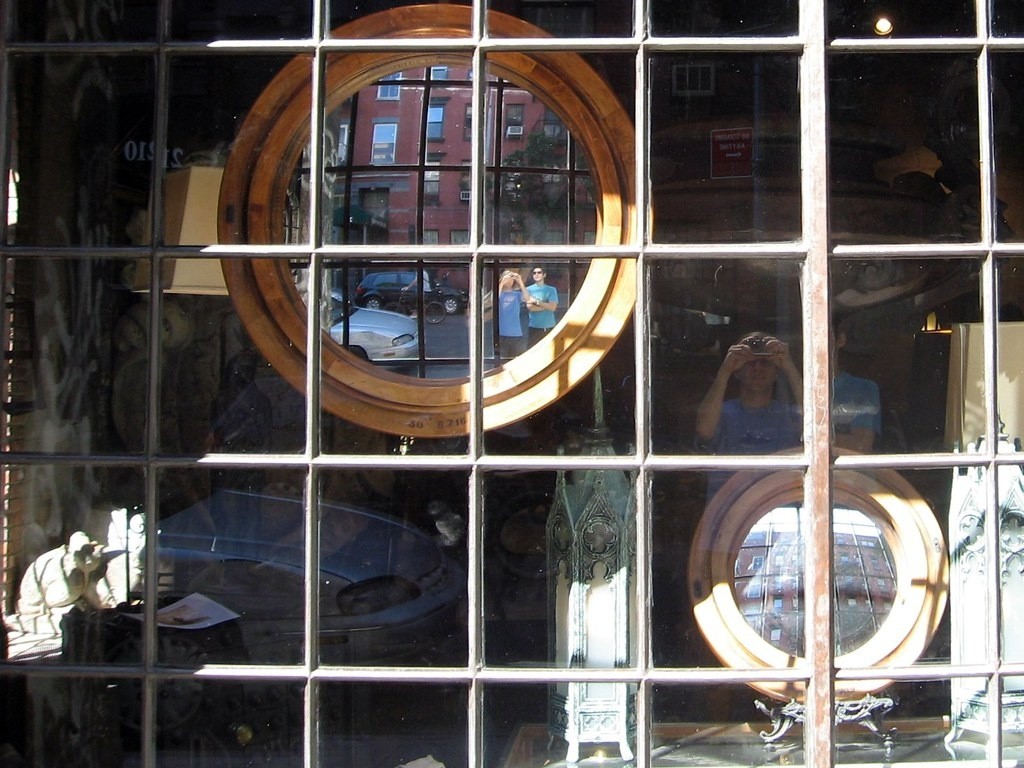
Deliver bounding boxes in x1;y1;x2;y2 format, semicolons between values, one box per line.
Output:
78;452;467;746
319;291;427;365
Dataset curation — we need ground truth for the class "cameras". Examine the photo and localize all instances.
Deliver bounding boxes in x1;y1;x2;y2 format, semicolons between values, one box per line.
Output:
747;339;783;356
510;273;518;279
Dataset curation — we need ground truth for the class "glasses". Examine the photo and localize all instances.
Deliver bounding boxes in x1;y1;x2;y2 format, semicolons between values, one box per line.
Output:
532;271;543;275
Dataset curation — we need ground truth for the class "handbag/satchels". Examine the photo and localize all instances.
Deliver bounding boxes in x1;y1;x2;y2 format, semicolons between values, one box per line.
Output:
428;275;437;289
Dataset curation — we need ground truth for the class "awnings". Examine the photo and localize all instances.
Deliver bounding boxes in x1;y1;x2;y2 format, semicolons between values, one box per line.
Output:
332;206;388;287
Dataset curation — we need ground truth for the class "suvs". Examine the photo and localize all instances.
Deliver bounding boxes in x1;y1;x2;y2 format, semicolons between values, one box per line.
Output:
354;271;468;316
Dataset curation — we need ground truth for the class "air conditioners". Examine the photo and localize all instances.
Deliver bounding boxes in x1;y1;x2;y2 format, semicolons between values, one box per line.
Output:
671;63;715;95
506;126;523;140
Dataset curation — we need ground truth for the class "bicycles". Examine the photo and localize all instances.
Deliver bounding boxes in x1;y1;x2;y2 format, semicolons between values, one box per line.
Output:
382;289;446;324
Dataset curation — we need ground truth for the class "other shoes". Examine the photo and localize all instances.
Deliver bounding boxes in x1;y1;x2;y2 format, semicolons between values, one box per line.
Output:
409;315;417;319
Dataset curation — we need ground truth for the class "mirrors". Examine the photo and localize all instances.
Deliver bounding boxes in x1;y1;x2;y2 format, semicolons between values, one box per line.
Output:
690;444;950;702
218;3;654;439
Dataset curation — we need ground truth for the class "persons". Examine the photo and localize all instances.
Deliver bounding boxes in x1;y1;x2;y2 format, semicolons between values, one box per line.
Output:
402;267;432;319
525;266;559;350
696;305;881;520
496;270;530;357
